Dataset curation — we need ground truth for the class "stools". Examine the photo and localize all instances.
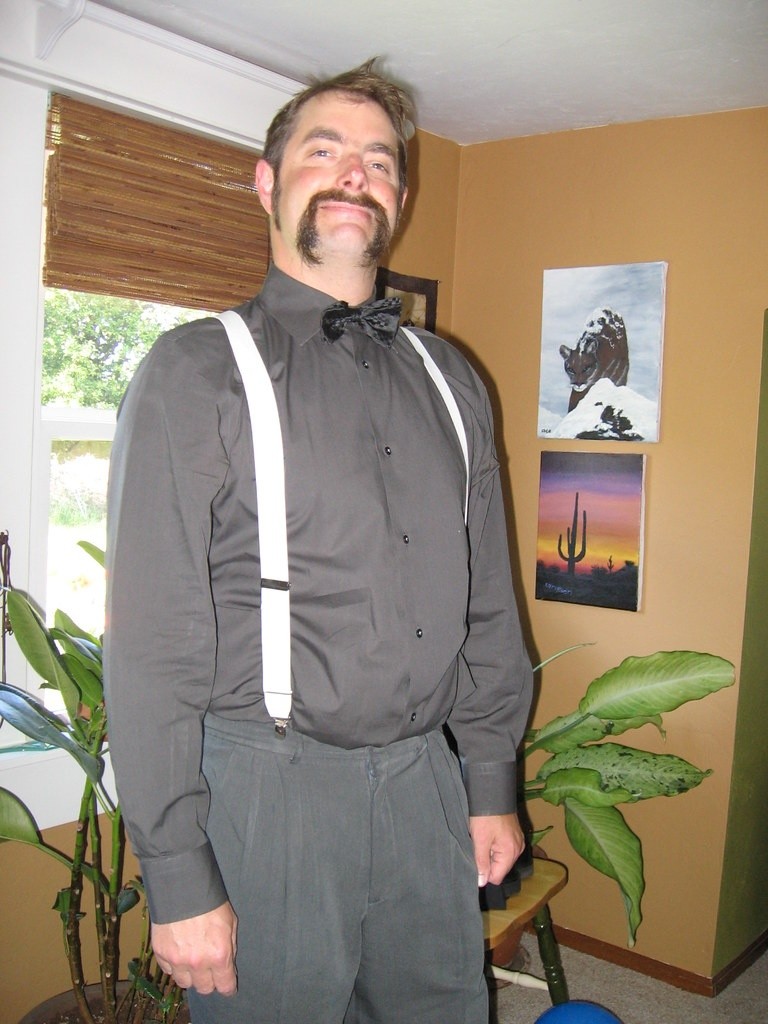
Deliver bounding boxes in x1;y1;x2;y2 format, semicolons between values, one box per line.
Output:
481;858;569;1024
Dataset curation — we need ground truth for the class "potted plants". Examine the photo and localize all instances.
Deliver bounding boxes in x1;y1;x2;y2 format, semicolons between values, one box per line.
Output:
479;641;737;988
0;541;198;1024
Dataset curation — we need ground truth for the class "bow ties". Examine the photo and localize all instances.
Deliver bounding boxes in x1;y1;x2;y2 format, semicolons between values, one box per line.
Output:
321;296;402;351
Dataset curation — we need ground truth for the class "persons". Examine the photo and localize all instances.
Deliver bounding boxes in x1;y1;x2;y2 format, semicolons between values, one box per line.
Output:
103;54;536;1024
